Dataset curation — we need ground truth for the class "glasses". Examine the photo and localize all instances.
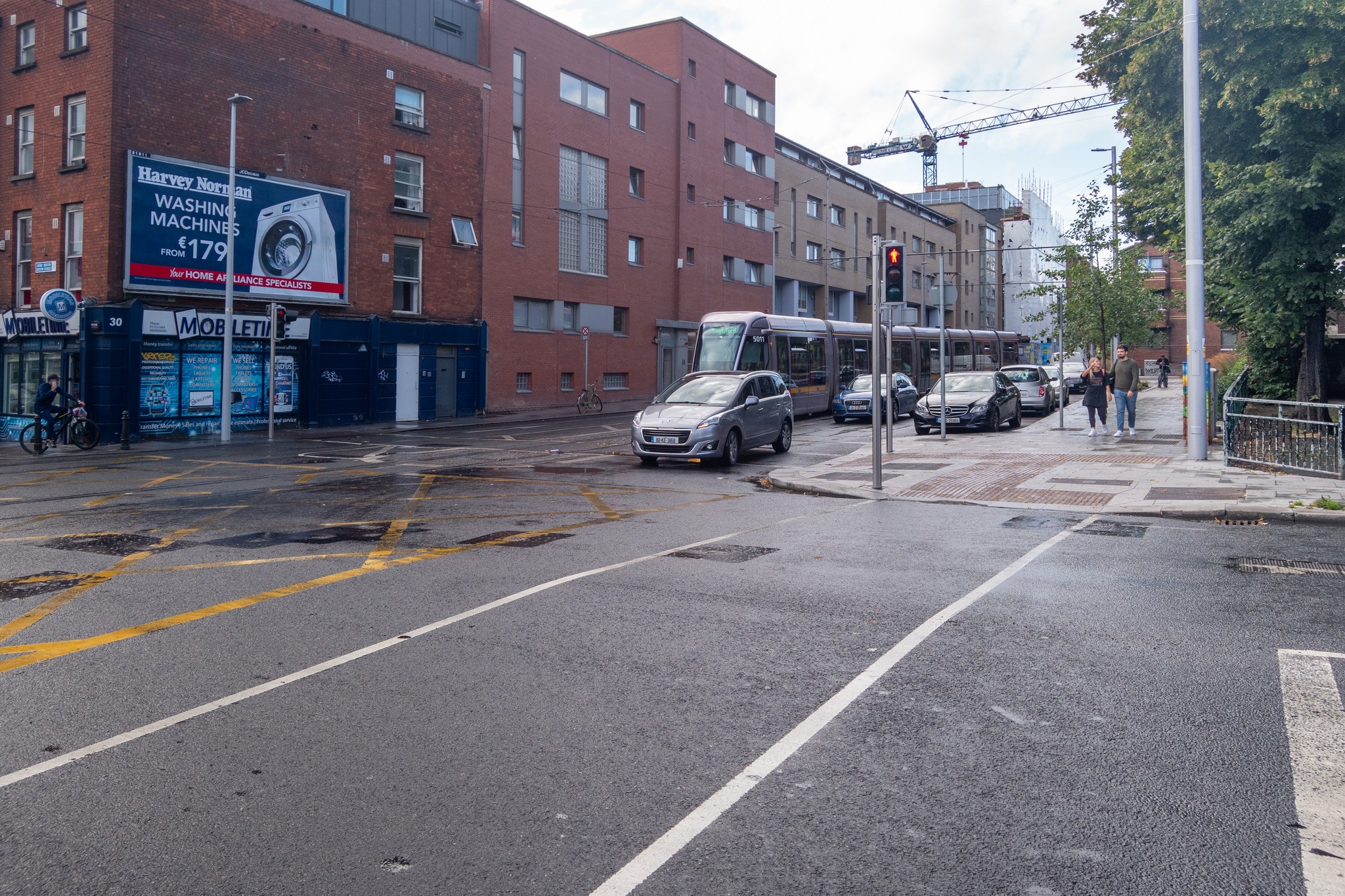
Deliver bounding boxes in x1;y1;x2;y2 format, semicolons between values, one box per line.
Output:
73;392;79;396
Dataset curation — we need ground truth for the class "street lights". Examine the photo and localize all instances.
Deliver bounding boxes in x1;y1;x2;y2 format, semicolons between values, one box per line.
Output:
1089;146;1123;343
219;91;255;444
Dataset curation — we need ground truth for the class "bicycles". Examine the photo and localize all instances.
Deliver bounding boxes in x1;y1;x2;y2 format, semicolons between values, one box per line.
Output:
20;403;101;454
577;380;603;413
1157;364;1171;388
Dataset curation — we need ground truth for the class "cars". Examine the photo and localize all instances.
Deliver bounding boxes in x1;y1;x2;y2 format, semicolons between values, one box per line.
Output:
998;364;1059;416
839;365;856;382
913;371;1022;434
809;365;830;384
1038;351;1089;406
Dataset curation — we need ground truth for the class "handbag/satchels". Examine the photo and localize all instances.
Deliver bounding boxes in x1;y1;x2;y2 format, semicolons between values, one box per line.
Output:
1110;376;1116;395
71;407;87;428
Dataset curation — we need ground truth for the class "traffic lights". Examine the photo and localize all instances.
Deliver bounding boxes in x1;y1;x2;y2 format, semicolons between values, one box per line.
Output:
274;306;286;340
883;243;905;306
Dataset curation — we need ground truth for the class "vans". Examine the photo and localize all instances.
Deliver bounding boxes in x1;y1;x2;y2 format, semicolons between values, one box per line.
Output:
945;355;998;373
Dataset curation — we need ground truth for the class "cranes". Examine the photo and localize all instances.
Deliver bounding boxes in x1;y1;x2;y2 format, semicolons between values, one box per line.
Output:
847;89;1128;188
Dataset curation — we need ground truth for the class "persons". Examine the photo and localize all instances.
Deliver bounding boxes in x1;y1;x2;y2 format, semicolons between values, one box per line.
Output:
1106;344;1139;436
1080;358;1112;436
34;374;85;448
1155;354;1170;388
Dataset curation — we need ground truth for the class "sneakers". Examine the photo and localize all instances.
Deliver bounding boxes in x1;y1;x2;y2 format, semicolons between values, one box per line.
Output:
1129;427;1136;435
1114;431;1123;437
1101;423;1108;434
1089;429;1097;436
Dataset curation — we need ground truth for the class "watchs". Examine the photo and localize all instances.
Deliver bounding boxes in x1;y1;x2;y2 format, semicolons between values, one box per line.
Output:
77;400;81;402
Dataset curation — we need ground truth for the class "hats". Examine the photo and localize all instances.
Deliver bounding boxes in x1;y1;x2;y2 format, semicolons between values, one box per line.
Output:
47;374;62;380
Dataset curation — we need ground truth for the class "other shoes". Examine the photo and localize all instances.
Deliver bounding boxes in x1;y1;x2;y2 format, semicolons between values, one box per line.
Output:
1158;385;1161;388
46;439;57;448
1166;385;1167;387
53;427;59;432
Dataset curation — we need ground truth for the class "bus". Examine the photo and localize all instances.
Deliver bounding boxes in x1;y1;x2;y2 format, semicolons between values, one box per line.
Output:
785;347;809;385
689;310;1020;416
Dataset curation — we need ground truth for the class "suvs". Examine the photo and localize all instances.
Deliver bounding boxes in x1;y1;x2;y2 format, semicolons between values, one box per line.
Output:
830;372;920;424
630;369;794;467
